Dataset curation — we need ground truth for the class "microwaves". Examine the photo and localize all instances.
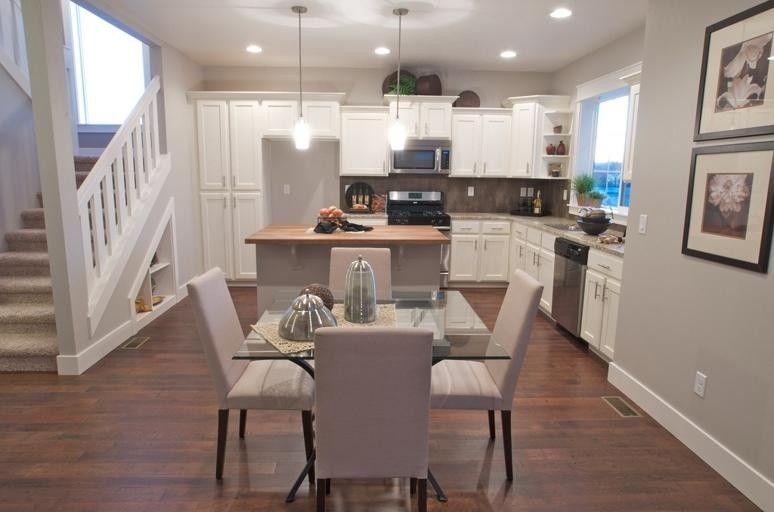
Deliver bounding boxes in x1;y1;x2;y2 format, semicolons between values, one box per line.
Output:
388;140;450;176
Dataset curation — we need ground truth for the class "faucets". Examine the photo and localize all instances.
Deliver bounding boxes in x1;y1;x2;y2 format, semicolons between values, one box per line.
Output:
577;207;589;214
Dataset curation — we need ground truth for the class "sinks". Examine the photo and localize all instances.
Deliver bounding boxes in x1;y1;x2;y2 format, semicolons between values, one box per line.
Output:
544;223;583;232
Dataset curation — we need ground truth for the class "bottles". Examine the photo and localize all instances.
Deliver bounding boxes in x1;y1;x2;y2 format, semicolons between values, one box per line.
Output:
546;144;557;155
557;140;566;155
533;190;543;213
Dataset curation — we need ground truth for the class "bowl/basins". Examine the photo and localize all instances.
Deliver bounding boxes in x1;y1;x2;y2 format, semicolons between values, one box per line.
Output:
575;220;609;235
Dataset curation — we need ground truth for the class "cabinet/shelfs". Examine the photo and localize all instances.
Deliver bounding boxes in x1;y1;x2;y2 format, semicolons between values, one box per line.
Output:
512;102;535;179
389;101;451;139
450;111;512;178
340;111;389;177
537;101;574;180
261;98;339;141
509;222;527;277
197;99;262;282
526;228;555;315
580;246;622;360
449;221;507;283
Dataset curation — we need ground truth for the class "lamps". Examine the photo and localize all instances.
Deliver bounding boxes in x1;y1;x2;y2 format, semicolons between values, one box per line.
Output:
290;5;309;151
387;8;409;153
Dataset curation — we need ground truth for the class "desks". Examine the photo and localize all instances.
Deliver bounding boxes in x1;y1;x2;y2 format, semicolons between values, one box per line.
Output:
244;223;451;317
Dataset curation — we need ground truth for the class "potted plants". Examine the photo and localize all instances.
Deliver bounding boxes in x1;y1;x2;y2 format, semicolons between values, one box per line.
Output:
588;191;607;208
388;74;442;96
569;173;596;206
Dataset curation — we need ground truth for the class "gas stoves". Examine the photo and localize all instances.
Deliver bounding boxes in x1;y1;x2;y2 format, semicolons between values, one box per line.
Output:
386;190;450;231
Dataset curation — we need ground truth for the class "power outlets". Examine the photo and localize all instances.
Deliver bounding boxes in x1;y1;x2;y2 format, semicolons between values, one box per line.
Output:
692;371;707;399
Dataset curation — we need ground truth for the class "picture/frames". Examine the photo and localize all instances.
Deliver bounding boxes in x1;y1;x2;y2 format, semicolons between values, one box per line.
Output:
681;140;774;274
692;0;774;142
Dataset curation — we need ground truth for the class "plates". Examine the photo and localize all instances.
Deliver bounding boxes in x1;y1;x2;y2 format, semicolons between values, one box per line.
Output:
510;210;551;217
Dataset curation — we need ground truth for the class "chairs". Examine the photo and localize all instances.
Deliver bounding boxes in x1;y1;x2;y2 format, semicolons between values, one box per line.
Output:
328;247;393;300
429;268;544;482
313;325;434;512
187;266;315;484
